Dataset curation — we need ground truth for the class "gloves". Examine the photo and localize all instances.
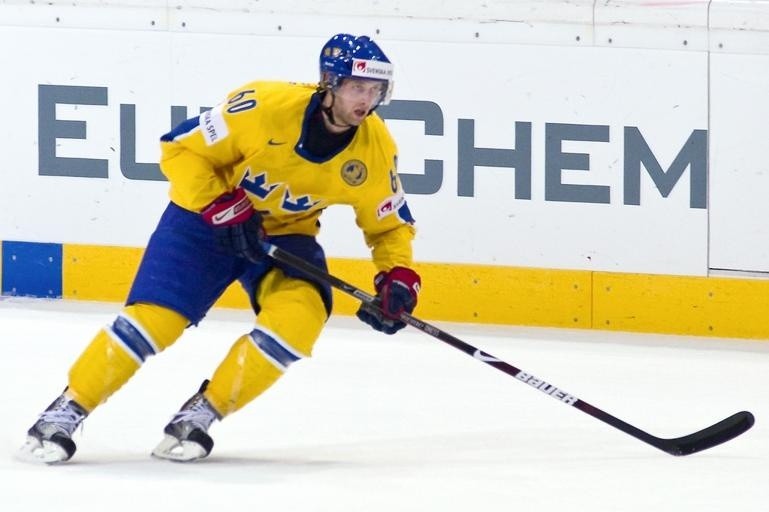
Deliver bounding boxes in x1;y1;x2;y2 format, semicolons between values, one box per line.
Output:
201;188;266;264
356;267;421;334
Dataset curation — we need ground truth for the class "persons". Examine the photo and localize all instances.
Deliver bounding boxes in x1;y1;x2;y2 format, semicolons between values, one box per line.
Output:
28;34;422;460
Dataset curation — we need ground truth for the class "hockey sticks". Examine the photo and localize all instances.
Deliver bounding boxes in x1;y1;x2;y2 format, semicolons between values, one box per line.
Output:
259;239;754;456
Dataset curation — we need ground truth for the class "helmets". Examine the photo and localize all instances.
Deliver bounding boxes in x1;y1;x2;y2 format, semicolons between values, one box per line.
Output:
320;34;393;106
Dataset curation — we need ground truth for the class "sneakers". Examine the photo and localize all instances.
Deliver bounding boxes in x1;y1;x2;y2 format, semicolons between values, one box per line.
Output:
165;393;217;453
29;395;88;458
